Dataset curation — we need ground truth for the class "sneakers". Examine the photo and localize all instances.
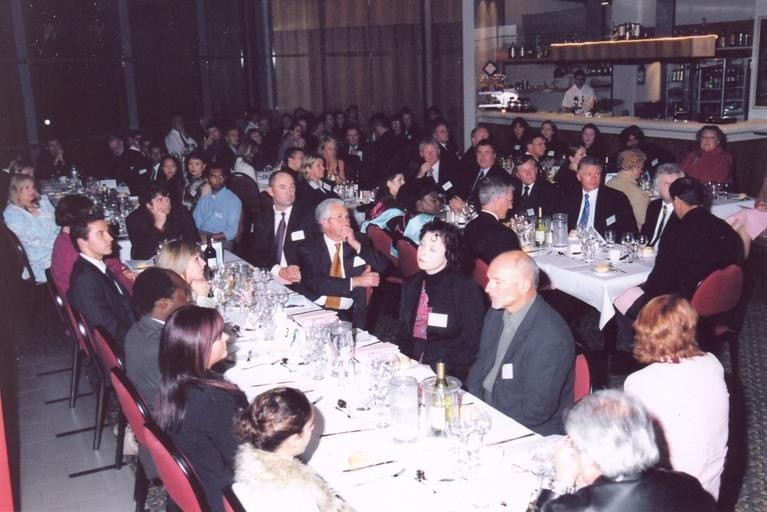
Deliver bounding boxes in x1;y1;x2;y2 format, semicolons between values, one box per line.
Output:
122;426;140;458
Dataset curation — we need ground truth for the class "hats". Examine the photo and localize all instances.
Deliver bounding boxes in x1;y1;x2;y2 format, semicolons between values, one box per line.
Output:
395;175;443;208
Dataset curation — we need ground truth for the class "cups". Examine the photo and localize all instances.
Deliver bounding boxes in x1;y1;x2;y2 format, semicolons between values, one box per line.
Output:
390;377;419;444
552;213;569;248
420;376;463;438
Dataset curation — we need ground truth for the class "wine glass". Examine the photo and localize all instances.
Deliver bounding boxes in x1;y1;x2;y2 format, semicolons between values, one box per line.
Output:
575;227;648;265
213;263;354;380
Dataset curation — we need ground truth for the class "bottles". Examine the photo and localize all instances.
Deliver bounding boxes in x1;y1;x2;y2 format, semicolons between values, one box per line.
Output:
342;167;363;200
205;235;216;280
509;42;516;58
591;63;609;73
671;32;753;87
535;207;546;247
66;164;127;234
520;40;526;58
432;360;454;437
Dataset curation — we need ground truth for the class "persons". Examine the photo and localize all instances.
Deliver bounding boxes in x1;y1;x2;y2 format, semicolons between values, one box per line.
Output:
577;123;608;159
538;119;564;159
621;297;733;511
553;143;589;189
462;250;576;436
4;152;354;511
614;176;746;361
107;107;555;383
606;125;661;240
683;124;735;199
524;387;720;511
637;160;688;252
560;71;598;115
556;154;640;244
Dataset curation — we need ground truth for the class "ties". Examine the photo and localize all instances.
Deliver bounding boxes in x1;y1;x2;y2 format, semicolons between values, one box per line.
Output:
105;268;118;292
324;243;343;309
522;186;529;200
474;170;484;189
152;170;155;181
179;133;188;148
578;194;590;229
649;204;667;247
272;212;286;264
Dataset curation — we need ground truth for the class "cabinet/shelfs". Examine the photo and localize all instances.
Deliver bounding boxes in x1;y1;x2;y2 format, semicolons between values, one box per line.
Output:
697;45;752;123
503;58;614;117
